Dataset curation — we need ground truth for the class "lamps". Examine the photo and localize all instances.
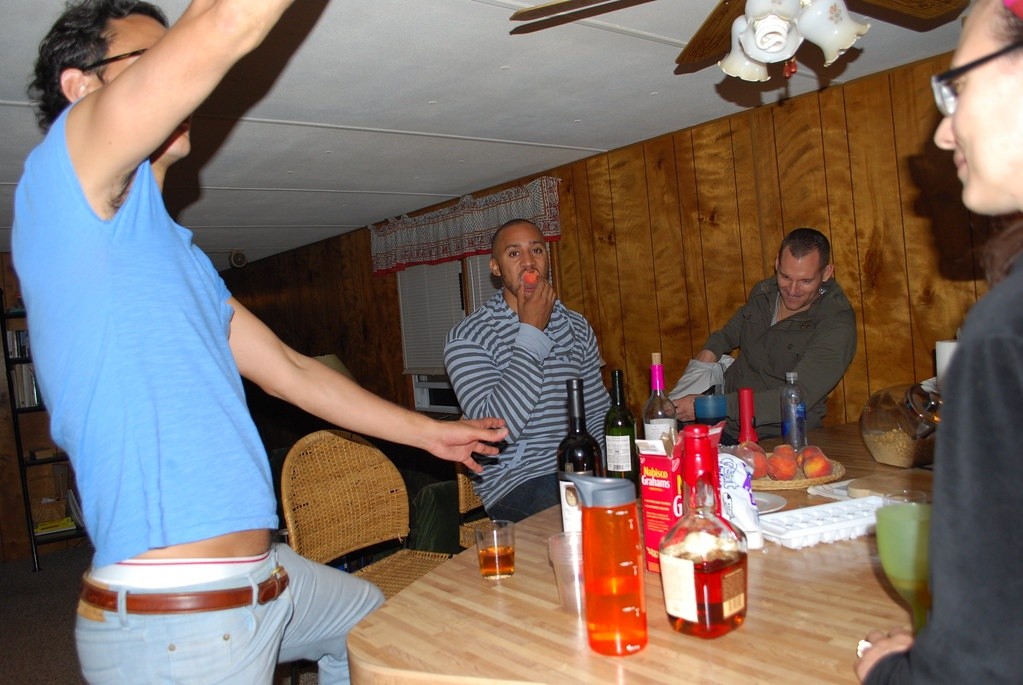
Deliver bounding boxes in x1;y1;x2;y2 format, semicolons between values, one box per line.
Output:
718;0;871;83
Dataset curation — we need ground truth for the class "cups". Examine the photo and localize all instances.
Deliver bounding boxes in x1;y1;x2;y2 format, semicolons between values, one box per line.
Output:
695;395;727;427
475;521;515;581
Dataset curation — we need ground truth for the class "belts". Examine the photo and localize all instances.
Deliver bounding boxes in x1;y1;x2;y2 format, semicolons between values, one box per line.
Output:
79;568;289;615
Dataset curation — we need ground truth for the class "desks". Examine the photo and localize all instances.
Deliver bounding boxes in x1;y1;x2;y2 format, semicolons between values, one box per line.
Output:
345;418;939;685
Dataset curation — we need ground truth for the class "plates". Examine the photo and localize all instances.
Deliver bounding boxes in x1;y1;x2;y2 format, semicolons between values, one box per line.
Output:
752;492;787;515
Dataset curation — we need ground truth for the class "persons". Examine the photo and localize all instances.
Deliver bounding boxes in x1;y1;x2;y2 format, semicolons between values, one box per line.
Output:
443;218;613;522
12;1;509;685
856;1;1023;685
671;228;857;441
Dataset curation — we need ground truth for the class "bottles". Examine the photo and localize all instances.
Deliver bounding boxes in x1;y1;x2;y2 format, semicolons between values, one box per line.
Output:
642;353;677;440
556;380;604;537
603;370;639;499
563;474;648;657
659;425;748;641
779;373;808;454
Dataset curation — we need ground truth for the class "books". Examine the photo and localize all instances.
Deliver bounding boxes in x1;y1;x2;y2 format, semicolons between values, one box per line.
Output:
29;447;52;459
6;329;42;407
35;489;85;535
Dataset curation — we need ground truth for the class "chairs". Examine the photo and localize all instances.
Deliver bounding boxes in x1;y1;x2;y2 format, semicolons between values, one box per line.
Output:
280;414;500;602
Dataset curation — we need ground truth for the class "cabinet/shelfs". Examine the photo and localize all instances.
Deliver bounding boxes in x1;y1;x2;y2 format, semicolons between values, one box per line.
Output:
0;288;88;573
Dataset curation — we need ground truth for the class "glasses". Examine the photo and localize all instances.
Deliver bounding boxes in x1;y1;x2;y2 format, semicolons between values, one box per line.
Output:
930;38;1023;117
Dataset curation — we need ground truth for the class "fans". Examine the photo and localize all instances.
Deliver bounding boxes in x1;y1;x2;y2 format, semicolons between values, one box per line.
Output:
510;0;972;67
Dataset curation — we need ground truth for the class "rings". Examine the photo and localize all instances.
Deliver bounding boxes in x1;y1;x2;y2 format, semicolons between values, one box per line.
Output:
857;639;870;657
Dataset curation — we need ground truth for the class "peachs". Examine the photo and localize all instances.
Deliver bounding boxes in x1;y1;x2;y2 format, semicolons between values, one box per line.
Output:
521;271;539;289
735;441;833;480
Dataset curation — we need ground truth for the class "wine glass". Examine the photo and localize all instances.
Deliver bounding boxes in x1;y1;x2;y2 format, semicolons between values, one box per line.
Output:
874;503;932;639
